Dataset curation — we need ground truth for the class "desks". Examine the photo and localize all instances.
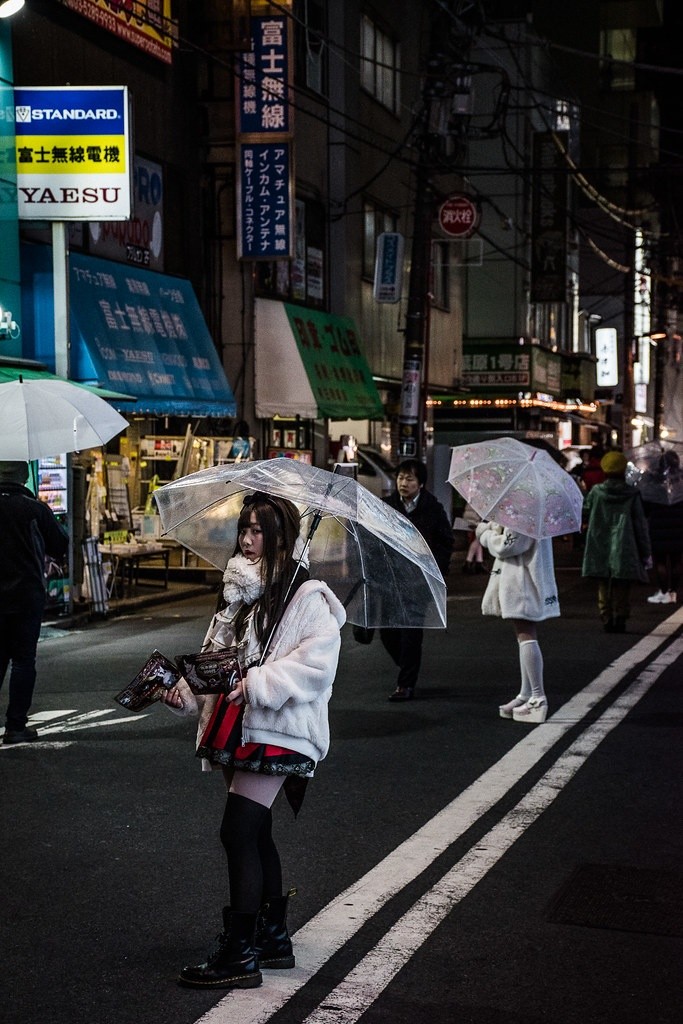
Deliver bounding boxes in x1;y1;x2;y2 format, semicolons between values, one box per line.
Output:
101;546;172;597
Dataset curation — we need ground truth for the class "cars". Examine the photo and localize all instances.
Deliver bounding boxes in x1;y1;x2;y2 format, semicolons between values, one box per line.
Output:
330;441;398;501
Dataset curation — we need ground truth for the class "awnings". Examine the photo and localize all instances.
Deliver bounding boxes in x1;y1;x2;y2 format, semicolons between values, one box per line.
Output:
18;244;237;420
0;368;137;404
253;296;386;422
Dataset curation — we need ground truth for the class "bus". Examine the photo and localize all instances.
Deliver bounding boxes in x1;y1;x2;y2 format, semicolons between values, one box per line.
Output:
433;397;619;451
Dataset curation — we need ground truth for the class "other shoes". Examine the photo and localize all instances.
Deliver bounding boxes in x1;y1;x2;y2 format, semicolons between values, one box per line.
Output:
3;727;37;743
388;686;414;701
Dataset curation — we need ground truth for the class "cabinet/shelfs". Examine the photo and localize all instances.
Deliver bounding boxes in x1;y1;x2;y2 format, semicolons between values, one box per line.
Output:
139;434;251;516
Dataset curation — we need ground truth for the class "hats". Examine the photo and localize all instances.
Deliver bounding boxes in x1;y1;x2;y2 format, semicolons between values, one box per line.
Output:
599;450;626;473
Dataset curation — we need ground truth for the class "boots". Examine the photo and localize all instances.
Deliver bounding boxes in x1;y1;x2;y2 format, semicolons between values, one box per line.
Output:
461;561;477;575
256;889;296;969
178;903;265;988
476;562;489;572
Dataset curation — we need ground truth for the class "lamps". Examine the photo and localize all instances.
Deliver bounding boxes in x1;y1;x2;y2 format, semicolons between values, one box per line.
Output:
380;417;391;451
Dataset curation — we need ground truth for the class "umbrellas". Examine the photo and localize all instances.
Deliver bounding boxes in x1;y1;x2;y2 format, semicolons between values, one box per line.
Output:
153;455;448;670
0;374;130;497
622;437;683;505
445;437;585;542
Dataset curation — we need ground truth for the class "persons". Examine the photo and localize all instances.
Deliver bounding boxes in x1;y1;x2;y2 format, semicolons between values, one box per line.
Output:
358;458;456;703
568;449;683;635
160;491;346;990
467;517;562;724
0;460;70;744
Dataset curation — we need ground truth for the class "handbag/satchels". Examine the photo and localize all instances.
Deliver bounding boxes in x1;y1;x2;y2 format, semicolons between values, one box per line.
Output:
352;579;377;644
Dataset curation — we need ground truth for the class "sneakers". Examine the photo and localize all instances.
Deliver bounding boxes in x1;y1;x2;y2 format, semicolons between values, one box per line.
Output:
646;589;677;604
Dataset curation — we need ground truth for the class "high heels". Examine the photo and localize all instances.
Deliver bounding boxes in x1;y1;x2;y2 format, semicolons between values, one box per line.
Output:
499;693;529;719
512;696;548;723
